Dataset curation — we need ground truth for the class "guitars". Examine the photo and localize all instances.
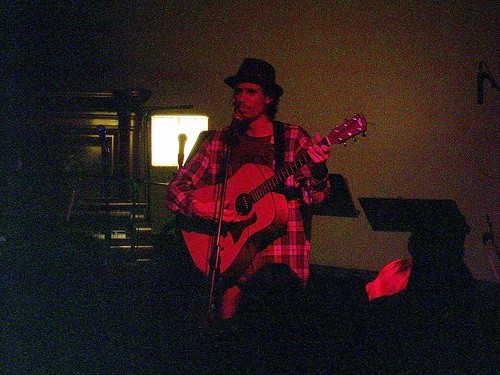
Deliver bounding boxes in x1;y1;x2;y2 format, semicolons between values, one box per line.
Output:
179;112;367;277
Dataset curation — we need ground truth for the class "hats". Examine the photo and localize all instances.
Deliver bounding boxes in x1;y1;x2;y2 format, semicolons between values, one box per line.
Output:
224;58;283;96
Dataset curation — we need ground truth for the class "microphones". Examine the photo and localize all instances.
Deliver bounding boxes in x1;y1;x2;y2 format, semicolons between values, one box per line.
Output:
477;62;484;104
95;124;108;153
226;113;242;146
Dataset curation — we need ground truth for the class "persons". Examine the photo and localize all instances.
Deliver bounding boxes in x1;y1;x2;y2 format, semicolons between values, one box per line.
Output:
167;58;331;325
